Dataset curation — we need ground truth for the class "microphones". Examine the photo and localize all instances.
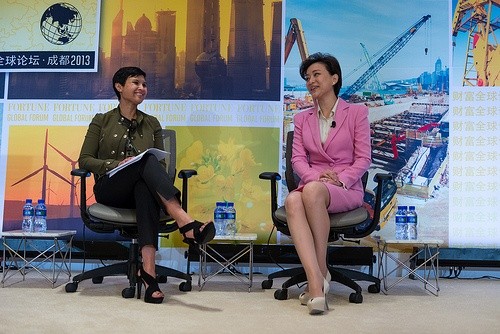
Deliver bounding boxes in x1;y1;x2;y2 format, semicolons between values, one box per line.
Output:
332;121;336;128
132;119;134;121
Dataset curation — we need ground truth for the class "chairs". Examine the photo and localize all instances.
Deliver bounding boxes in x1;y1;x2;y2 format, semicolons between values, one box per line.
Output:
259;131;392;303
65;130;197;298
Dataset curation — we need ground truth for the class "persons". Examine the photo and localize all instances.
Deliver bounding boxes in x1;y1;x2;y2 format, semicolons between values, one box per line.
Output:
78;67;216;303
285;52;371;315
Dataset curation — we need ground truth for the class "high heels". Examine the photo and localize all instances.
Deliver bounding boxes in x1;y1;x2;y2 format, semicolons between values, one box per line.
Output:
180;219;216;258
299;270;332;304
308;278;330;314
135;265;164;303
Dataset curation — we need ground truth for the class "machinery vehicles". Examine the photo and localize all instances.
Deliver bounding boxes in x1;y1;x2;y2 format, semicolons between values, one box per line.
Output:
284;18;313;111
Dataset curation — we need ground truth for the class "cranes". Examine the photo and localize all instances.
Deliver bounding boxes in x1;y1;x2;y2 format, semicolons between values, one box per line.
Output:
451;0;500;88
340;13;432;101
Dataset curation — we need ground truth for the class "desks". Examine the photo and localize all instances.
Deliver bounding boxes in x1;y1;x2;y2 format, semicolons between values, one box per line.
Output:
0;229;77;288
198;234;258;292
378;239;444;297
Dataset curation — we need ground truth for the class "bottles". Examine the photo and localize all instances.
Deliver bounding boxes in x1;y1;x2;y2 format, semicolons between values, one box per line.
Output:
214;202;227;238
22;199;35;236
395;205;417;239
34;199;48;234
226;202;236;238
